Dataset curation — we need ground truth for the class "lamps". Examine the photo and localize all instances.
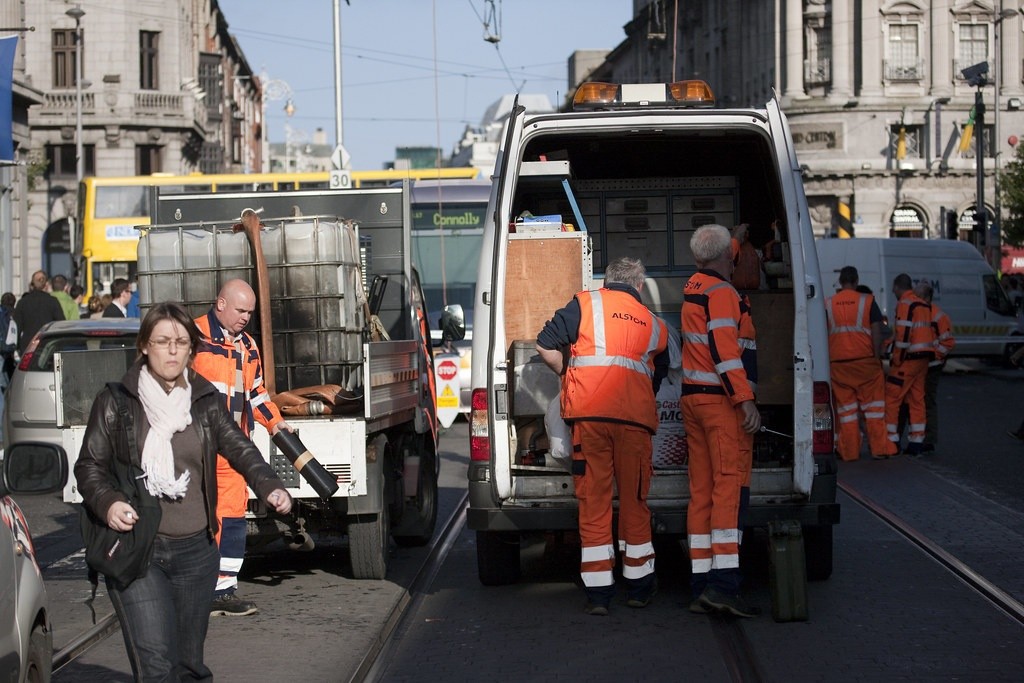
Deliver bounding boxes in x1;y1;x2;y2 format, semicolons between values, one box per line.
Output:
938;96;951;105
180;78;208;102
848;96;859;106
862;163;871;170
1011;98;1021;107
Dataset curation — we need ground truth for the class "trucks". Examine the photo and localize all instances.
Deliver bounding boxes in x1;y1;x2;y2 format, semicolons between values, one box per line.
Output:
53;177;459;581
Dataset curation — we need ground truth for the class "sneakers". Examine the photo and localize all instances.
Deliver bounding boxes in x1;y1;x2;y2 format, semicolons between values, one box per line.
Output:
584;587;608;615
626;573;656;610
689;571;758;618
211;586;258;616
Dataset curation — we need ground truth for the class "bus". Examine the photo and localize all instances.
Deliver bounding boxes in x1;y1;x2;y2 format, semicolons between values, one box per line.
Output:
69;168;479;307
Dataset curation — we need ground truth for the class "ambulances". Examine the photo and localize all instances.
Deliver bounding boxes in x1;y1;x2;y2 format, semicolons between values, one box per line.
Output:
432;75;842;587
817;238;1024;367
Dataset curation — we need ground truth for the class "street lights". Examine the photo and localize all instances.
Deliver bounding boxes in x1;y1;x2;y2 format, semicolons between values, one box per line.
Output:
66;8;87;182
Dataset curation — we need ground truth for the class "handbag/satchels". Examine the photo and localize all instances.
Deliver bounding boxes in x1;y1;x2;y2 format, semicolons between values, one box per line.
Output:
80;380;162;584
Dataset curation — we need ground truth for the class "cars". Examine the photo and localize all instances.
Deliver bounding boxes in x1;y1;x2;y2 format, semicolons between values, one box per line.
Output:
0;439;68;683
7;318;142;441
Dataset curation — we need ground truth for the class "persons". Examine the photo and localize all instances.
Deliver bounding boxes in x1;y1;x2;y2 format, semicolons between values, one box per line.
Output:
0;267;139;416
71;300;292;683
824;265;956;461
1006;346;1024;441
682;222;764;618
534;257;673;617
185;277;296;618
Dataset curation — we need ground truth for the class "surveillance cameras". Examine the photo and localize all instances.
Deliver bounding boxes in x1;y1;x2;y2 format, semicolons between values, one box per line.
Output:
961;62;989;80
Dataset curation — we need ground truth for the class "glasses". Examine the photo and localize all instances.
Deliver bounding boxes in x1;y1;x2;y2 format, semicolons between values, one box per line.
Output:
152;336;192;352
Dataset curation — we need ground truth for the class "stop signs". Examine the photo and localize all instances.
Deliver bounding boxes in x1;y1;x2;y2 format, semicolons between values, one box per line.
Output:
438;360;458;380
